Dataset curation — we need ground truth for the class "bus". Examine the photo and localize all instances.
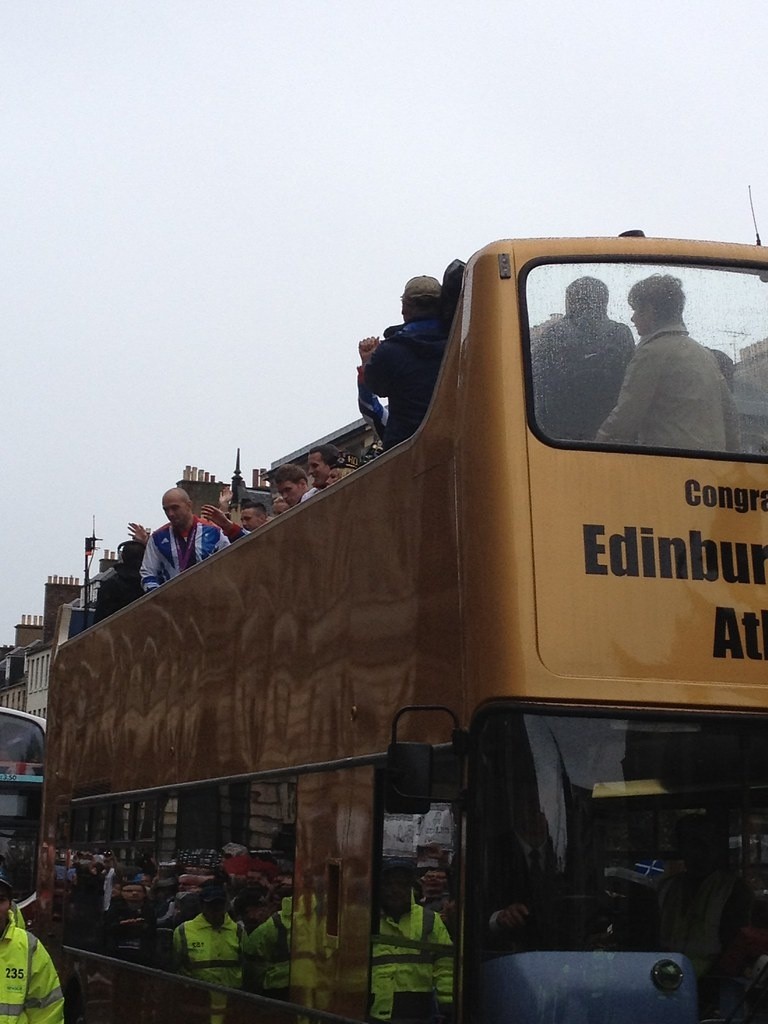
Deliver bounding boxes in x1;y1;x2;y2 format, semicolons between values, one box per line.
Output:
588;772;768;898
40;237;768;1023
0;707;45;930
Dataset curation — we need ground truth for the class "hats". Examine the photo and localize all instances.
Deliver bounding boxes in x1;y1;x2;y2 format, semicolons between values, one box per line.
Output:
122;541;145;566
201;885;226;903
404;275;441;306
327;452;359;469
0;870;13;888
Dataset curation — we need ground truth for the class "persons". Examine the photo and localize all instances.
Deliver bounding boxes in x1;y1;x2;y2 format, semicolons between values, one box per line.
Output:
528;273;741;452
54;845;458;1024
487;783;629;950
644;838;752;1023
356;275;447;453
92;444;362;621
0;876;64;1024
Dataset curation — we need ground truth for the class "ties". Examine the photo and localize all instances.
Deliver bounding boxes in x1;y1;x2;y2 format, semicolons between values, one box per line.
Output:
529;850;541;875
